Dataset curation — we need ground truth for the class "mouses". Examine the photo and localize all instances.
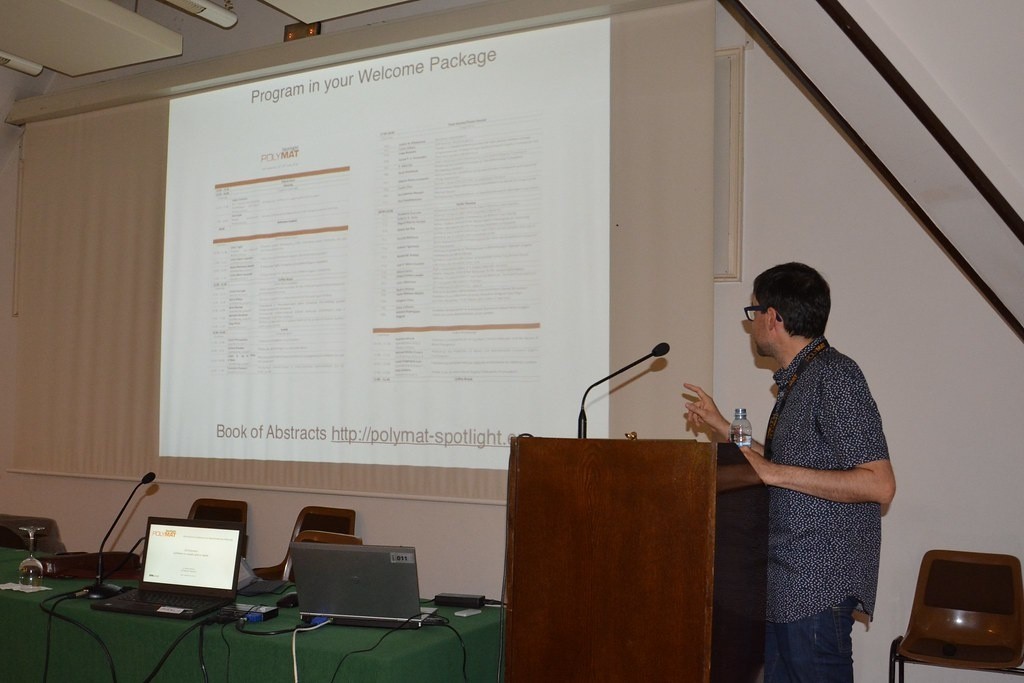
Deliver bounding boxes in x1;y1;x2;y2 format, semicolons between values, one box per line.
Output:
277;591;299;608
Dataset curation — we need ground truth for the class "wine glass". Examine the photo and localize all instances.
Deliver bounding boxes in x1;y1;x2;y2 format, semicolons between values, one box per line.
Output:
17;526;47;586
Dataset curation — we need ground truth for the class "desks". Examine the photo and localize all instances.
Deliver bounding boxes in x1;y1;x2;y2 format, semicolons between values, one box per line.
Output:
0;546;506;683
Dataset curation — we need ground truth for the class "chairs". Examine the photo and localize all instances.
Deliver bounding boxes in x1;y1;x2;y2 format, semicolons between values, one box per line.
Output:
253;506;356;579
888;550;1024;683
187;498;248;557
280;530;362;583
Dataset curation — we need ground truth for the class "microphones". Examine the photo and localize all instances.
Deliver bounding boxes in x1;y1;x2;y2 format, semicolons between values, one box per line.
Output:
577;343;670;439
83;473;157;598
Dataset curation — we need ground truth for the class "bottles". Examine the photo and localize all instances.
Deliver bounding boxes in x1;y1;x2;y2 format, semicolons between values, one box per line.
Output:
728;408;752;448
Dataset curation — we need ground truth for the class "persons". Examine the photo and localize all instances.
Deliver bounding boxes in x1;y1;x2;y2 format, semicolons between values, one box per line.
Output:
684;261;896;683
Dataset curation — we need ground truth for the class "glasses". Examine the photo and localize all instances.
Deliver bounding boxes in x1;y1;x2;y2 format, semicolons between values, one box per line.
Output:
743;306;783;324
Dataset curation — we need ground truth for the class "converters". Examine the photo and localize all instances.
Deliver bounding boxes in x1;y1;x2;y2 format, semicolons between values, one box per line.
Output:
435;593;485;609
252;606;278;620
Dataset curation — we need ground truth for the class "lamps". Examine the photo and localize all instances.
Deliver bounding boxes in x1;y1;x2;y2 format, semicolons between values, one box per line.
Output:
0;50;44;76
165;0;238;29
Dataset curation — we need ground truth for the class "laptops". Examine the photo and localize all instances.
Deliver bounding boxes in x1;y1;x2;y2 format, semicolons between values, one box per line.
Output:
289;541;438;629
91;516;245;621
237;556;290;594
